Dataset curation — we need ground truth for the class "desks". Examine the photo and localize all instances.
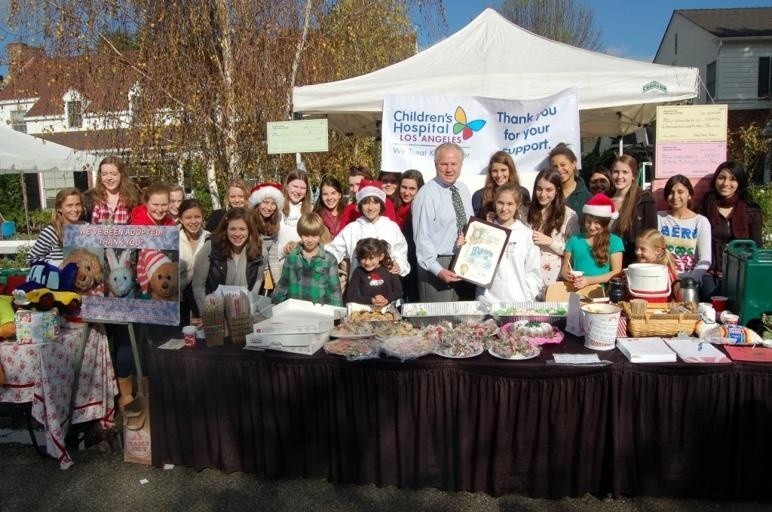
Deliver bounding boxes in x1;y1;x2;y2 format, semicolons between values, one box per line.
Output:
1;317;122;465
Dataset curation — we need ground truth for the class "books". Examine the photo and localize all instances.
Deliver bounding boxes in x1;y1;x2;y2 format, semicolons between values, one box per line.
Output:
616;336;733;365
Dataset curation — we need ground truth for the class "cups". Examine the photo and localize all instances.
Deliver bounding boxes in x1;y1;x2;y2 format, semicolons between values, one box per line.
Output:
724;314;739;325
183;324;198;348
711;296;728;310
571;271;585;281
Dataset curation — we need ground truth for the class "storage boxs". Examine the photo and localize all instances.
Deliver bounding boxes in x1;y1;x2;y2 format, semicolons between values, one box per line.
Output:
115;372;153;466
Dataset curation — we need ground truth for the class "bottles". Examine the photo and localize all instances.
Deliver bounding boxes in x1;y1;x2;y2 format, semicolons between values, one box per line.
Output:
608;278;625;302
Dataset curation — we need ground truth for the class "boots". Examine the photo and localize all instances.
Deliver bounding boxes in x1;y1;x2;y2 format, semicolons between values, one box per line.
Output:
118;377;134;408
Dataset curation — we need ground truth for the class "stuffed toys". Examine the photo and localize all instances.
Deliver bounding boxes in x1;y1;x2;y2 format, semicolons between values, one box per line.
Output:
103;247;136;299
62;246;104;297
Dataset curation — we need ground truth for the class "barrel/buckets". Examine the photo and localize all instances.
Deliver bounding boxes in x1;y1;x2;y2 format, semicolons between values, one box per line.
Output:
1;220;16;239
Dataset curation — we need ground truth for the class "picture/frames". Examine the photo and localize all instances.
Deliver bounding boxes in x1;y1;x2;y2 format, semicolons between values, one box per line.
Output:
452;215;514;289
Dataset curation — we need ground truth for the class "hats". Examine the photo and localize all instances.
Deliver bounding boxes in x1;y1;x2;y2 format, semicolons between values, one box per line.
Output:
355;179;386;205
582;192;620;220
247;182;285;215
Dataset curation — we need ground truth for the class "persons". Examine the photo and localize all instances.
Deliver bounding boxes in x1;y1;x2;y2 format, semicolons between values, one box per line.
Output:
128;141;616;343
25;186;91;272
623;226;683;304
655;173;713;291
560;191;628;296
611;155;658;270
693;161;765;296
83;156;154;419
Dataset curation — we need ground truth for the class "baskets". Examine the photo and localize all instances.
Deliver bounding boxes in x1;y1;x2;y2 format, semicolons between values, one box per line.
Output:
617;300;701;337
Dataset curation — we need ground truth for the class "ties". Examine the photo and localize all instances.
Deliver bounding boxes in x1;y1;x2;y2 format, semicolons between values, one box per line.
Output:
450;185;467;235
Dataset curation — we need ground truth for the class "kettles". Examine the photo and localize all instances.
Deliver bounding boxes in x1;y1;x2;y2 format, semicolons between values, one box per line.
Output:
671;276;700;304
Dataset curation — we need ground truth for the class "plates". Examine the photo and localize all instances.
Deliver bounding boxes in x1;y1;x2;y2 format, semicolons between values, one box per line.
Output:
462;328;500;338
433;346;484;359
331;330;380;340
489;343;542;361
